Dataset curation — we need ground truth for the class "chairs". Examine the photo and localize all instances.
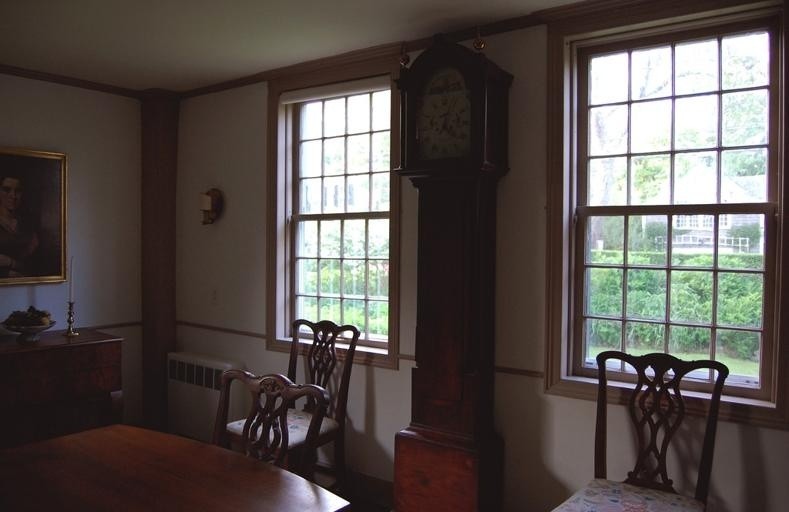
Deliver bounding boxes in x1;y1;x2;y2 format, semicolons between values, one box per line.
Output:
549;350;729;512
210;370;330;483
223;320;360;497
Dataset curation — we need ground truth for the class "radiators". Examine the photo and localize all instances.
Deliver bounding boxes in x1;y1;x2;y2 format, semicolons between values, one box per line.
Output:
165;351;243;443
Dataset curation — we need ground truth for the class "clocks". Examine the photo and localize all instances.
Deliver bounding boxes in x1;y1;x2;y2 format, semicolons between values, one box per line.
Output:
391;28;513;511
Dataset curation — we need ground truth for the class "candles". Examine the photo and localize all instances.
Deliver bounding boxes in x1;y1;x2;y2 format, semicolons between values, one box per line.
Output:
69;256;75;302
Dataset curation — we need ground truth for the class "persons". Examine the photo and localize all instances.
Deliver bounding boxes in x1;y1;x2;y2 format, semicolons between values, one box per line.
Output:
0;168;44;278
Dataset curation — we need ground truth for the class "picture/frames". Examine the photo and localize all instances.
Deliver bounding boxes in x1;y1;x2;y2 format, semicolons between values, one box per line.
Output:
0;146;67;286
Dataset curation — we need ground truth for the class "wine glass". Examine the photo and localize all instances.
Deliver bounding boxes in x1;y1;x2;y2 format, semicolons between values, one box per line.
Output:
0;320;57;343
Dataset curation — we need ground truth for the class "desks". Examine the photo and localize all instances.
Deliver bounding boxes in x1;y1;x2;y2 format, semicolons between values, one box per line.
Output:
0;422;351;512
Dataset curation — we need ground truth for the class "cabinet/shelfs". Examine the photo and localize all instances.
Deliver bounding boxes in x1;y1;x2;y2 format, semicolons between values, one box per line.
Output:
0;328;125;450
